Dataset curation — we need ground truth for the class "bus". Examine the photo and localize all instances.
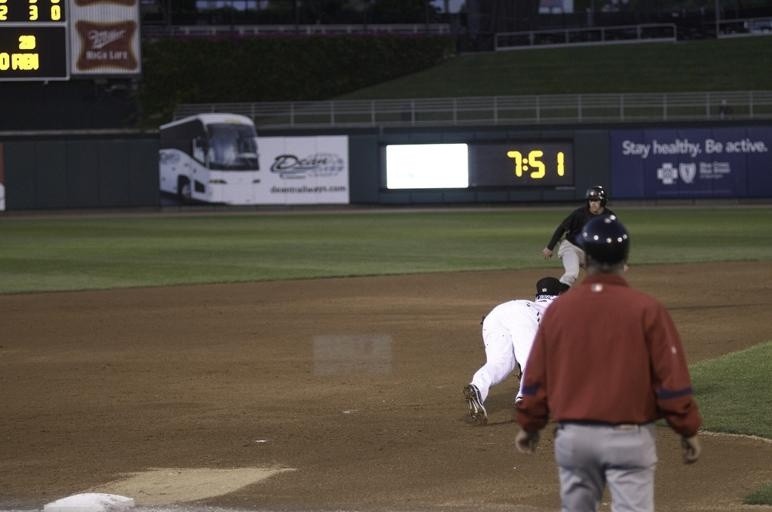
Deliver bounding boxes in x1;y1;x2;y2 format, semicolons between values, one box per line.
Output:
158;110;266;209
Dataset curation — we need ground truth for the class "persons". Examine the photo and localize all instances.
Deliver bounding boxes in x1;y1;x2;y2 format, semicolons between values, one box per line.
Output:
462;274;566;426
541;184;630;292
513;213;704;510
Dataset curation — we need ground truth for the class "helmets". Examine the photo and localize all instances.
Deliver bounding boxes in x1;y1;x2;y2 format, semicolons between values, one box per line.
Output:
584;184;609;207
577;214;630;264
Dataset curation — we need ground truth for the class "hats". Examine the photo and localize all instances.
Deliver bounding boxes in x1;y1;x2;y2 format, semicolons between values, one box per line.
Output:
536;276;570;295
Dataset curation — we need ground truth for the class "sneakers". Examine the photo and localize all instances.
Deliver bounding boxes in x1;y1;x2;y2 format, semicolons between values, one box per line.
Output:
462;383;489;426
